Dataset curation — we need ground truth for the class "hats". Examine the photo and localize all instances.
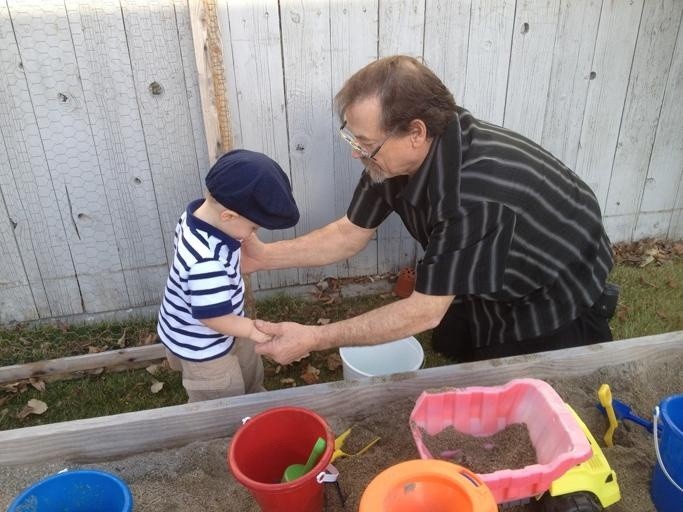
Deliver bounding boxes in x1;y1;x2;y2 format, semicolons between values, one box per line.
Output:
205;148;300;230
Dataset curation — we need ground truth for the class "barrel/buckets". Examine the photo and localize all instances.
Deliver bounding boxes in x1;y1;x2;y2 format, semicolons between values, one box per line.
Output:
225;407;340;512
339;336;426;381
649;393;683;512
4;468;133;512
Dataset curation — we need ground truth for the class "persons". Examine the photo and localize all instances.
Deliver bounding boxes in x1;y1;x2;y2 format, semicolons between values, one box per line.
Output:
154;149;312;405
235;54;619;368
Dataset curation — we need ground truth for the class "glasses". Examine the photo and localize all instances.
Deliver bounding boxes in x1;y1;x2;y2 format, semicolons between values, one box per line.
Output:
338;120;395;161
